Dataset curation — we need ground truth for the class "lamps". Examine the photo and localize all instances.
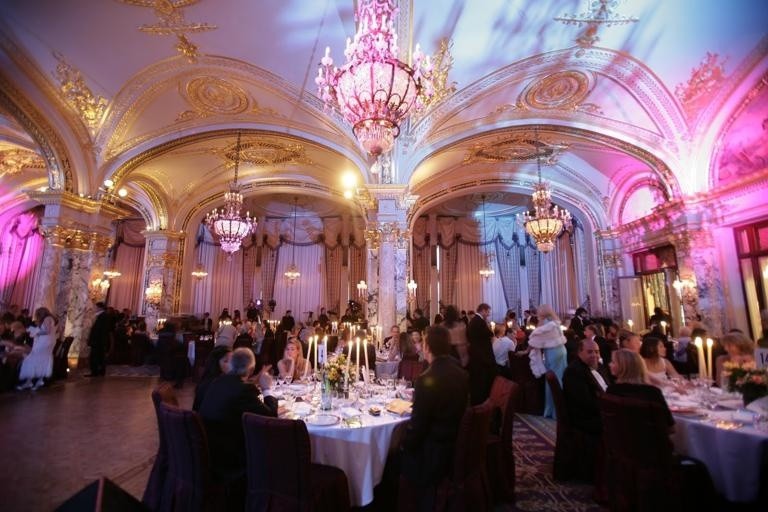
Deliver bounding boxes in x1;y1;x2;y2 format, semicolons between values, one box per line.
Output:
364;222;413;250
97;178;129;207
90;269;122;306
204;131;257;255
284;197;302;283
672;273;699;307
314;0;456;174
478;195;495;282
515;126;574;253
144;279;162;307
356;280;367;299
191;221;209;282
407;279;417;298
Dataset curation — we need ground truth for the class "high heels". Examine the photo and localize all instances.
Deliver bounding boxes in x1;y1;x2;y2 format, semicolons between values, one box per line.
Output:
15;380;45;392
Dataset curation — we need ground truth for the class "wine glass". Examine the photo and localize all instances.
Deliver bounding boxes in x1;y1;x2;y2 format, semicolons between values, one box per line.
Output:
651;372;768;427
216;320;345;346
266;371;411;425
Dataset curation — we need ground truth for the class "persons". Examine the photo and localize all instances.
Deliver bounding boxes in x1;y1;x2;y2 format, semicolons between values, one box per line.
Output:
0;302;57;393
85;302;146;377
157;302;377;422
375;325;470;512
529;303;768;421
379;303;539;406
561;337;726;512
